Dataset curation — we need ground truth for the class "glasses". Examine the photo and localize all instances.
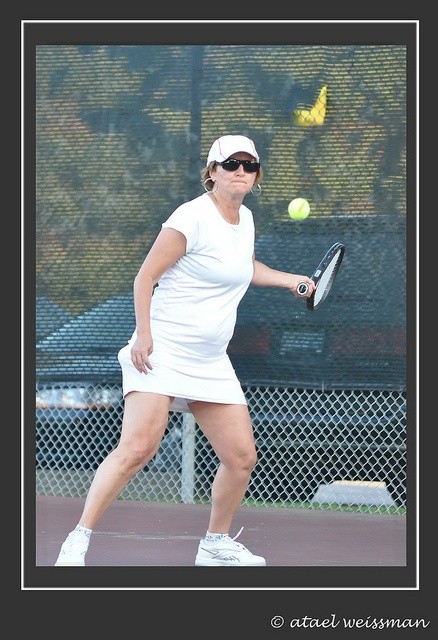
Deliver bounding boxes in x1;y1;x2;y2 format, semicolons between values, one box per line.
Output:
215;158;261;173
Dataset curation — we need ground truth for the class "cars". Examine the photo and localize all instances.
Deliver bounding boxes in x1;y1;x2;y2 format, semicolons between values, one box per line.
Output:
35;291;181;471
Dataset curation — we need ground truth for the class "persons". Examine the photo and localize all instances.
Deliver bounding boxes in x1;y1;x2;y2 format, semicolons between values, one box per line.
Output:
53;135;317;569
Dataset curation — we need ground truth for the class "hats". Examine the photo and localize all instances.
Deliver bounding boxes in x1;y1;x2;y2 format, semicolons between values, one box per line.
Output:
206;135;260;168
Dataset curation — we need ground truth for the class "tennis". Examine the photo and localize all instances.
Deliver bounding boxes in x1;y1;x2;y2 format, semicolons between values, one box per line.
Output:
288;198;310;220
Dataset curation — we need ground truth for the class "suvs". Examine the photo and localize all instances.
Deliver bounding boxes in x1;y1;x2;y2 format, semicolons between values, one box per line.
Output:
226;211;408;507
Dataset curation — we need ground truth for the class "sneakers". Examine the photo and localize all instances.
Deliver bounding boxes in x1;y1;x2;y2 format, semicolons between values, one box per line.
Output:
194;527;267;567
53;529;90;567
